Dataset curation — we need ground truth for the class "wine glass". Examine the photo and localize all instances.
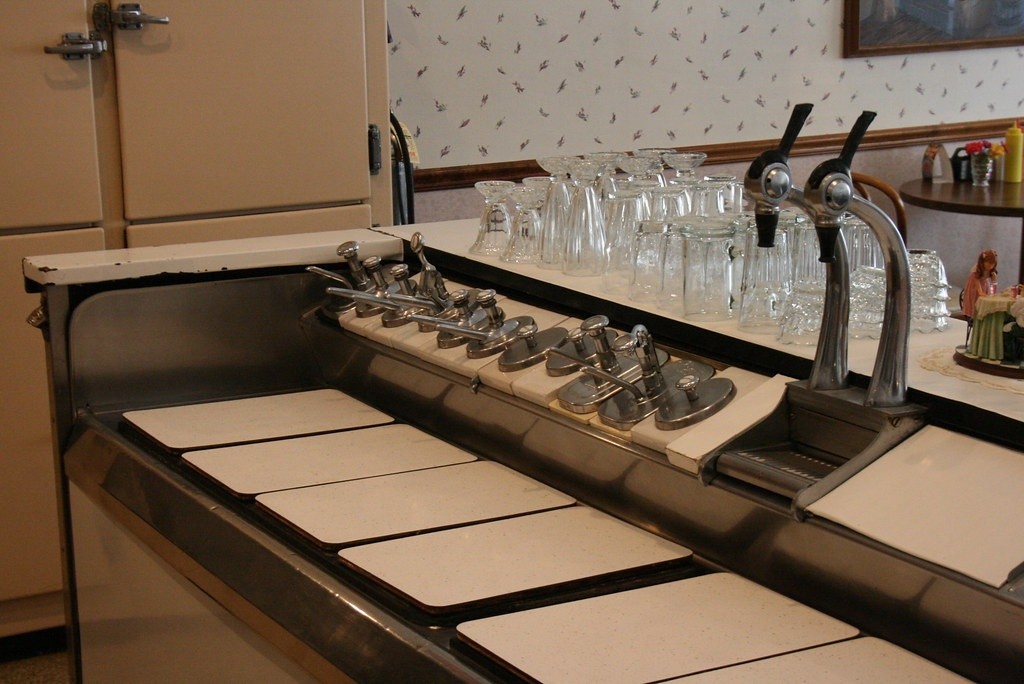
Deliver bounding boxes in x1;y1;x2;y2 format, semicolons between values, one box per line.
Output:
467;150;749;276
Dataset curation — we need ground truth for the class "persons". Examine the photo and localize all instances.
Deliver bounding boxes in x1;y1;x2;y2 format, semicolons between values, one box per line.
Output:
963;248;1001;321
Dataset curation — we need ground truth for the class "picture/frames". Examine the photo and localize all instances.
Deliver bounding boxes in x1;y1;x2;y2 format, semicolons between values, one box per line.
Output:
842;0;1024;59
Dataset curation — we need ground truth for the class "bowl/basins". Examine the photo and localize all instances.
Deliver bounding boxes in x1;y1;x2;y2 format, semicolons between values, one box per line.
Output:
776;249;952;345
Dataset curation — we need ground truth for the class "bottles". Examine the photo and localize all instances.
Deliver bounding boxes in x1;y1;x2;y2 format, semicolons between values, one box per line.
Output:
992;117;1024;184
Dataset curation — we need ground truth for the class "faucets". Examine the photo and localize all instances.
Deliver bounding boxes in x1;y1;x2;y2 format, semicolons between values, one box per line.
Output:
306;230;715;433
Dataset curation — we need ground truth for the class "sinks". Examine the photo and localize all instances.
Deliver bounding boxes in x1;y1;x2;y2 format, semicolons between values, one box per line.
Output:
119;386;976;684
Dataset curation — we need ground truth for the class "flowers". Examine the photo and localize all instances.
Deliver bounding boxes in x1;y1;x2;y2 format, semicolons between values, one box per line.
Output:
965;140;1008;177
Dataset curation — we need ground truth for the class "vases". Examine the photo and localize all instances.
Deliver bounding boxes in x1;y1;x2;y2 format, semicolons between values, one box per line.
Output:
971;154;994;187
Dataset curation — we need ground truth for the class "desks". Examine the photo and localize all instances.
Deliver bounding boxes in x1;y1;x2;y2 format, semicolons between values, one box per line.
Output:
899;178;1024;292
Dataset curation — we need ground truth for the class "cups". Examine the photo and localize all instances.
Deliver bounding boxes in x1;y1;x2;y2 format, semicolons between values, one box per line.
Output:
630;208;885;337
